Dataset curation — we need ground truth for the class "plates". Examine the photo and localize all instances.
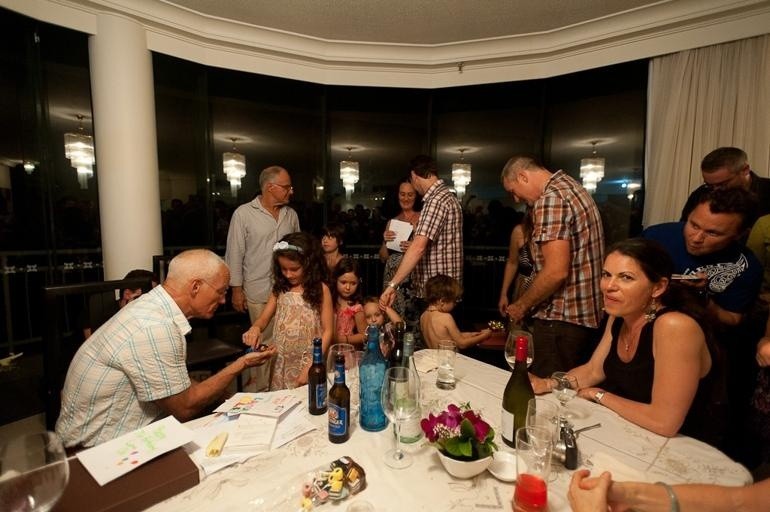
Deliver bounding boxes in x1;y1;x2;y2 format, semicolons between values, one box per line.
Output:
486;450;528;484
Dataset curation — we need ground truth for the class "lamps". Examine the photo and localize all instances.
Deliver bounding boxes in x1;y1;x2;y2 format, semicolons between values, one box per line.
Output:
339;145;361;201
61;112;97;192
221;135;247;199
449;147;473;201
579;136;608;197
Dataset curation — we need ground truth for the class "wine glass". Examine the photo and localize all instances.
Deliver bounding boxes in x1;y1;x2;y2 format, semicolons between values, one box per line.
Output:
504;330;535;372
381;366;417;470
515;372;580;511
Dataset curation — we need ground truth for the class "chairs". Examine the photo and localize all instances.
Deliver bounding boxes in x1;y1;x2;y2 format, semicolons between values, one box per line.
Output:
444;261;542;373
45;253;254;431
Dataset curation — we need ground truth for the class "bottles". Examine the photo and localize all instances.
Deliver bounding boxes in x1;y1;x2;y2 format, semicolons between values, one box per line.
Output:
307;328;328;415
359;324;389;433
329;353;350;445
501;337;537;448
387;321;424;444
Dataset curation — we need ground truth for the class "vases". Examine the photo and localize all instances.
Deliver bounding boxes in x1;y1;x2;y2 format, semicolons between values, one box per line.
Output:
437;442;495;480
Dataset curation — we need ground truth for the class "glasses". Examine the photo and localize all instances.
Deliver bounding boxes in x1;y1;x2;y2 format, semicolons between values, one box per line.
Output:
703;172;740;191
272;182;293;191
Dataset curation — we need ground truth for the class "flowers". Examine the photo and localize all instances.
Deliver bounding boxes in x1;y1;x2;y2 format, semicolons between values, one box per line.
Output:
419;394;501;460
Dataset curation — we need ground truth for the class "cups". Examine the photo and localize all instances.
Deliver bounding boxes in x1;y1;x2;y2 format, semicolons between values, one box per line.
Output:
435;339;457;391
327;343;357;389
1;431;70;512
